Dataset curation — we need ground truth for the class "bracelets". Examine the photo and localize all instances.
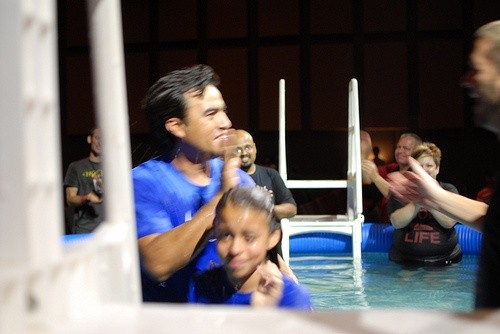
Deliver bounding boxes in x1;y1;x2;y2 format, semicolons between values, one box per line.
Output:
80;194;84;202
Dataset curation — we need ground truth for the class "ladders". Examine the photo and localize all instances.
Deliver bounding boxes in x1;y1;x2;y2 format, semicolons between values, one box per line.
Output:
278;77;364;286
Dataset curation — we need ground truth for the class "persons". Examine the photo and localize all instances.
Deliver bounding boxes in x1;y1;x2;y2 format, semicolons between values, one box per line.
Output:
194;186;312;308
372;147;385;164
64;125;104;234
387;21;500;310
388;143;463;268
132;64;256;304
360;131;423;224
237;129;297;270
